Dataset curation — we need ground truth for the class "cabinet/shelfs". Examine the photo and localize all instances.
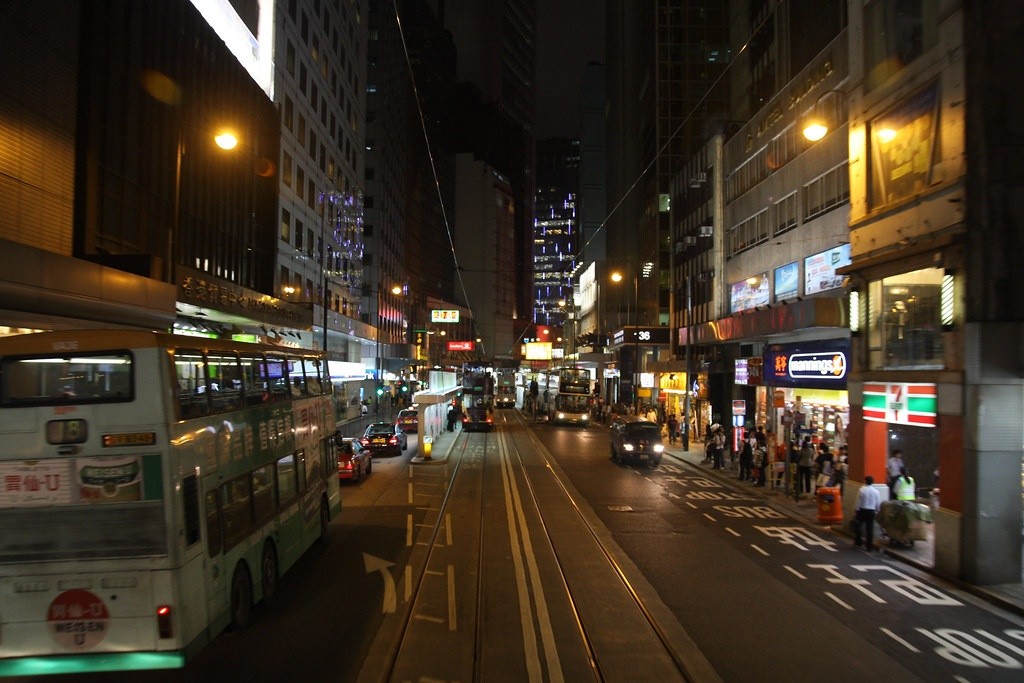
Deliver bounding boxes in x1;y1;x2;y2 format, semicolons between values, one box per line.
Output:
768;462;792;488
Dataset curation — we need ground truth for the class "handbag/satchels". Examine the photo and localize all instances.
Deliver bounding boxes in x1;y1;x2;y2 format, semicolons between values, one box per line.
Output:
815;474;831;487
707;442;717;451
848;520;861;534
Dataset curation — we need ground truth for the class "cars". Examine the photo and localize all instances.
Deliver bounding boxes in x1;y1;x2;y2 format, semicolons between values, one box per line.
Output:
337;435;372;483
362;421;408;455
396;409;418;432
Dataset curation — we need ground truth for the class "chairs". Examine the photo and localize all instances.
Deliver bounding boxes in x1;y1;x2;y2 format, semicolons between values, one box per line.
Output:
181;384;310;418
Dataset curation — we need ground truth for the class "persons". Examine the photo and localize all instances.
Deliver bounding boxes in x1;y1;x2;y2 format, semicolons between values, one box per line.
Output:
591;397;666;432
314;377;321;394
337;431;343;445
523;377;539;414
337;439;353;454
667;412;685;444
700;423;727;471
391;392;407;406
738;424;915;552
543;389;553;403
447;406;458;430
306;376;317;395
361;396;372;416
291;376;304;396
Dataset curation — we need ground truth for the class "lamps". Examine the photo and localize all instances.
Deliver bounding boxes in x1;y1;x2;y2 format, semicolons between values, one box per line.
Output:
803;89;849;143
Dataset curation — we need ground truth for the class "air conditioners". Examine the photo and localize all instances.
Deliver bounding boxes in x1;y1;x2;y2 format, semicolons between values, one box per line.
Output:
697;172;707;182
698;227;714;237
689;177;701;188
677;242;686;250
684;236;697;245
699;273;706;282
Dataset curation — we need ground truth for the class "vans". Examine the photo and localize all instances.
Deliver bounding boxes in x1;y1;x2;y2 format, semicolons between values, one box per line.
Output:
608;414;663;467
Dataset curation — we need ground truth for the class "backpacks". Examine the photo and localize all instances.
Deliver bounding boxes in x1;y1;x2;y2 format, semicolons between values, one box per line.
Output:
757;447;769;468
741;440;753;458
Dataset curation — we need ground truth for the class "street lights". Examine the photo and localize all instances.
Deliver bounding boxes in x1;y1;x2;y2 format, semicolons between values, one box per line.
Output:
610;259;640;416
556;294;577;369
374;274;404;413
171;120;246;265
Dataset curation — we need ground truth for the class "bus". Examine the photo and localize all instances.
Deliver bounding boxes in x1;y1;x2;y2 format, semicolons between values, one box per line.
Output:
457;361;497;432
543;366;594;427
0;327;343;683
495;373;518;409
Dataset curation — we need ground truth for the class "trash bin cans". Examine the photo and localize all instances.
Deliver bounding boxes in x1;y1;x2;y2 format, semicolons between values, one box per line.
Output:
816;487;843;524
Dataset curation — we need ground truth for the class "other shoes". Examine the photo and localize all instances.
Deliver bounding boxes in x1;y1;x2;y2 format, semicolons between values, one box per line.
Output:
855;542;861;546
868;548;872;551
